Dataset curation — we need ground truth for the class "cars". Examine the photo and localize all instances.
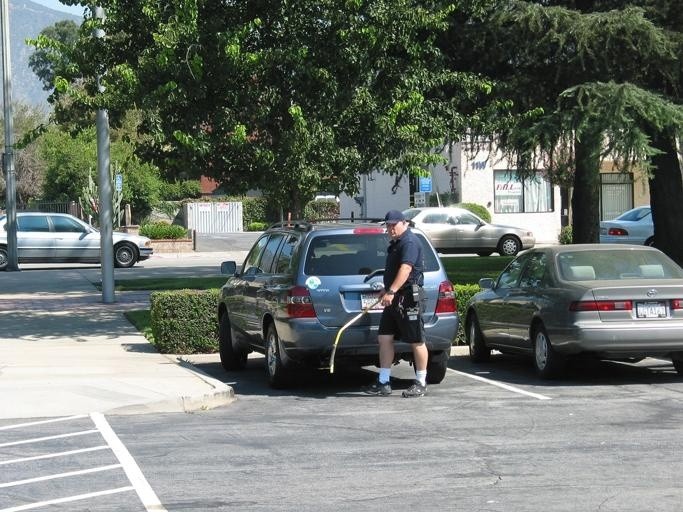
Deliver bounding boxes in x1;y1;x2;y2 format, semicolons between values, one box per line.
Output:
398;206;537;257
464;243;682;378
0;212;154;270
598;206;656;247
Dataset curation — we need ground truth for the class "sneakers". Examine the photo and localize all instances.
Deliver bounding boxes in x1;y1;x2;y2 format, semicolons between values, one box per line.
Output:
401;380;427;398
360;376;392;395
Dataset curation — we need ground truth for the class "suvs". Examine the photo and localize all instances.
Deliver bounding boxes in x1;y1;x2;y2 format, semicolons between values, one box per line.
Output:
210;215;459;389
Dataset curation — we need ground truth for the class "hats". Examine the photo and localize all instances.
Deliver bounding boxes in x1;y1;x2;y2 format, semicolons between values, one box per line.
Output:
380;210;405;226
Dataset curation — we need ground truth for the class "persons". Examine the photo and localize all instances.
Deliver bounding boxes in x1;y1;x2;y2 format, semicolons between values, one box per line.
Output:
357;209;430;399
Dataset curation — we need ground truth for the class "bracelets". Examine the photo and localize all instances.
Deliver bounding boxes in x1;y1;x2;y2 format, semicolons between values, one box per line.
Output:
386;286;397;297
